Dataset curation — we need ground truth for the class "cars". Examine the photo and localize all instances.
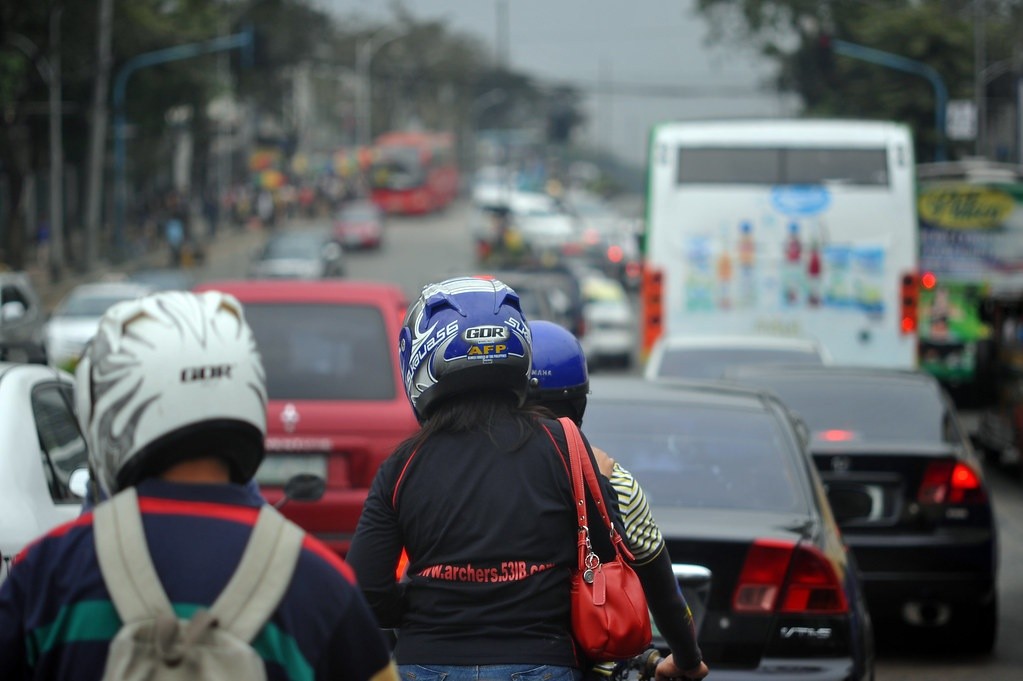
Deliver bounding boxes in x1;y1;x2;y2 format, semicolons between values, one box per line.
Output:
466;160;1000;681
182;278;415;569
42;201;409;371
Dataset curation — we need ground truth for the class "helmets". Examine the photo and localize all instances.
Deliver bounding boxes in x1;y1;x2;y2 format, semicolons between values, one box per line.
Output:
398;276;532;426
525;319;589;403
74;289;266;498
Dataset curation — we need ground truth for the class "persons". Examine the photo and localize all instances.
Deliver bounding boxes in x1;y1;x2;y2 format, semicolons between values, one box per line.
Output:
221;170;346;230
0;288;397;681
164;212;183;266
527;320;709;681
347;276;630;681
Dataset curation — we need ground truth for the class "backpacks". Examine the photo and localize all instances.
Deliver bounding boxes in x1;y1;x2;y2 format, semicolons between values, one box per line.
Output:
94;485;307;679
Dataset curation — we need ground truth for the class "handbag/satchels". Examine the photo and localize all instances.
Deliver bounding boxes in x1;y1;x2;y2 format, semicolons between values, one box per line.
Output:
557;416;653;660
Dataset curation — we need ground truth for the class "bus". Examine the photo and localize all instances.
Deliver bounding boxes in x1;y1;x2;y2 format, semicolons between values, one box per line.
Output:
649;118;916;369
358;131;459;213
